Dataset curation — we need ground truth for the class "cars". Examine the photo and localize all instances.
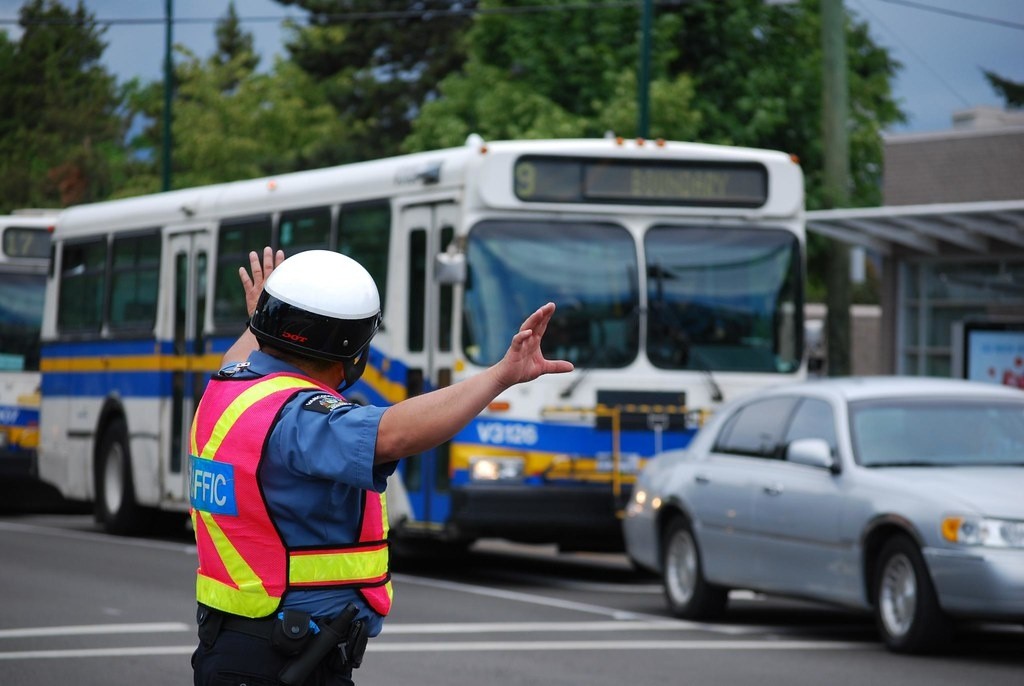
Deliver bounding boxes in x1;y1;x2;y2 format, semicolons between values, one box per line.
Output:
619;378;1023;658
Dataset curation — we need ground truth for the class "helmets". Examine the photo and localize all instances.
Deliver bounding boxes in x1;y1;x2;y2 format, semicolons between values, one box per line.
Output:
250;250;383;362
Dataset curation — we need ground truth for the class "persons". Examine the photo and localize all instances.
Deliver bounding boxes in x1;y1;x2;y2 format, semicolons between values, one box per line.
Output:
187;246;573;686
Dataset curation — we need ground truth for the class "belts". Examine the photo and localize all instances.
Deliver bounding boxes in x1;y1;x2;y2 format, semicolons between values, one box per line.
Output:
222;615;353;673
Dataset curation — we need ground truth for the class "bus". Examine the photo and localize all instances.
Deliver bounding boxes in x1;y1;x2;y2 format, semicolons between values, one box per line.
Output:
0;209;68;471
36;133;810;576
